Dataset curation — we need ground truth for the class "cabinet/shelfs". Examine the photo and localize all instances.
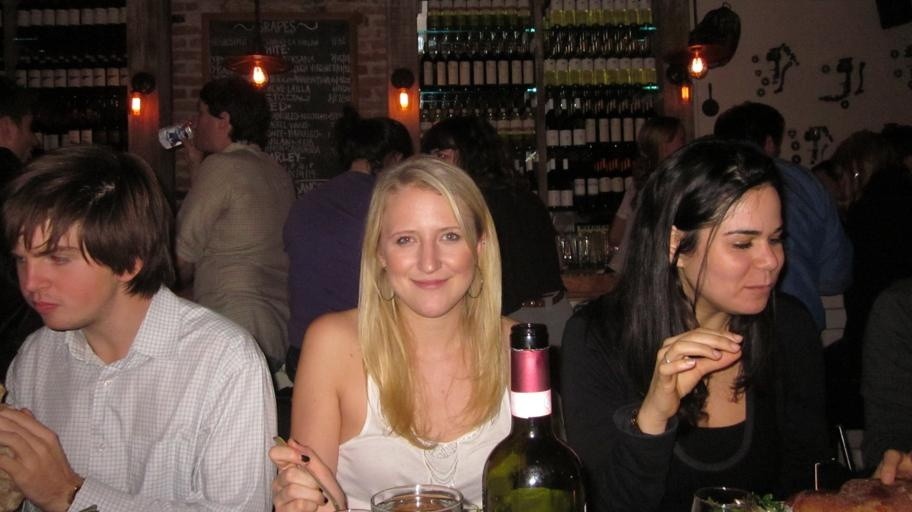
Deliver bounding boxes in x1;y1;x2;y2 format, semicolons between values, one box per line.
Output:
1;0;174;187
421;0;697;272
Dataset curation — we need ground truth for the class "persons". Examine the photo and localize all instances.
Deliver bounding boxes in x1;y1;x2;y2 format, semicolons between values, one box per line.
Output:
285;117;414;383
268;156;523;512
845;125;911;323
555;135;911;512
860;280;912;452
170;77;291;373
0;76;35;335
420;116;573;351
0;142;278;511
607;116;686;276
714;101;854;350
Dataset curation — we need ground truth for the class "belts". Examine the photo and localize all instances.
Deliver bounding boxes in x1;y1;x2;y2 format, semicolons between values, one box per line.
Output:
519;286;565;308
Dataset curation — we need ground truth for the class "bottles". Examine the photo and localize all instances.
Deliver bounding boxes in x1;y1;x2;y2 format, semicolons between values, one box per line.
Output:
156;121;195;148
421;2;662;211
0;0;128;152
481;321;587;512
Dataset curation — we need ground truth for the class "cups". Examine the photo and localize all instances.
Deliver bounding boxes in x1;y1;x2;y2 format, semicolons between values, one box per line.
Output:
576;236;589;265
590;230;609;274
371;484;480;512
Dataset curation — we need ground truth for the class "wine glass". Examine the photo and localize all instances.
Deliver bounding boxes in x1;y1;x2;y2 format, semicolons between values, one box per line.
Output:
690;487;756;512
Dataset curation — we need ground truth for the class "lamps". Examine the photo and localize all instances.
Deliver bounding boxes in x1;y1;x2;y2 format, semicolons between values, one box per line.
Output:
391;68;414;113
677;64;693;104
129;70;156;114
686;0;706;75
244;1;272;87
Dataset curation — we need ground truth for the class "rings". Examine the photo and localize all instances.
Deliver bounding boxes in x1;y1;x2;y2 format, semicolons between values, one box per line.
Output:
664;351;671;364
